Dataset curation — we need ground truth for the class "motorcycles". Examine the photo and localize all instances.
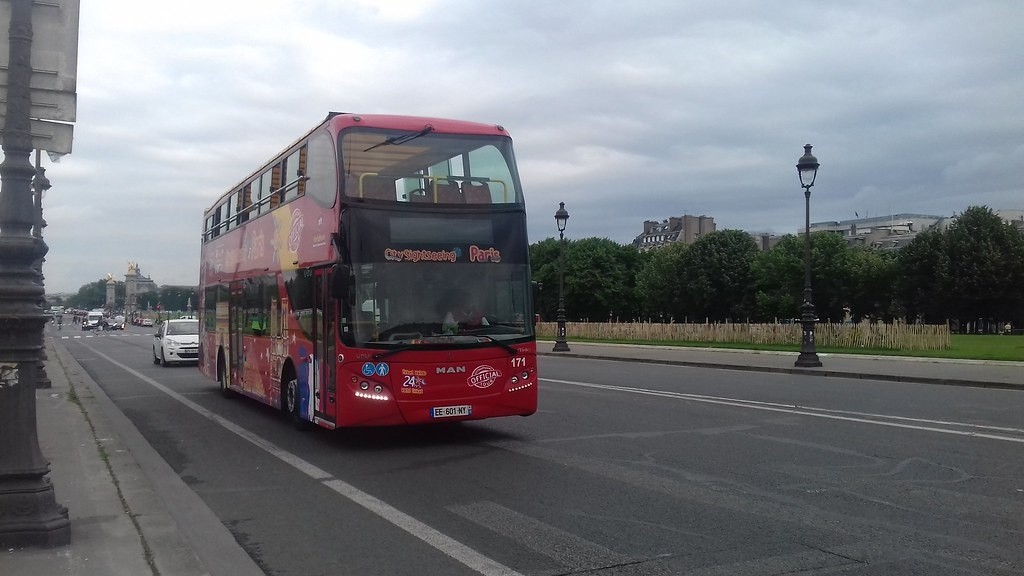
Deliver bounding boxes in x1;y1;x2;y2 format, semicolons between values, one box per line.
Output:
82;322;87;331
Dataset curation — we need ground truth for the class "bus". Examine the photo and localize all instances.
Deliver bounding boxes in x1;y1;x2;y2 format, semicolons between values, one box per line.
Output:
197;112;540;433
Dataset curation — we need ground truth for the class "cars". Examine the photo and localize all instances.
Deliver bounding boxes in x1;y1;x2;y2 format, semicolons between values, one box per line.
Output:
141;318;153;327
152;319;208;367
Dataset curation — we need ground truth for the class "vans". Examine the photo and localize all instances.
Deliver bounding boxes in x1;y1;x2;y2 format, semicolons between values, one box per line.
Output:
87;311;104;330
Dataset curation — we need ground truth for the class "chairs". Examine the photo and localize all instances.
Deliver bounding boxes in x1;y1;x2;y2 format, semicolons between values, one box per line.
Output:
346;173;494;204
358;312;376;339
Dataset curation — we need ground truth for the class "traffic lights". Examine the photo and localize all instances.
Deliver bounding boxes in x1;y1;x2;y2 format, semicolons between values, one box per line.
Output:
157;305;161;309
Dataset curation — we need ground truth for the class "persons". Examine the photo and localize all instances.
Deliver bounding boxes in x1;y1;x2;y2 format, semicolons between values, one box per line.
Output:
442;263;490;333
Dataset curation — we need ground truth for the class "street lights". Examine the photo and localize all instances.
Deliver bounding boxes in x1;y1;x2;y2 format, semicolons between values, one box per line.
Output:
552;201;571;352
190;287;195;319
794;143;823;367
